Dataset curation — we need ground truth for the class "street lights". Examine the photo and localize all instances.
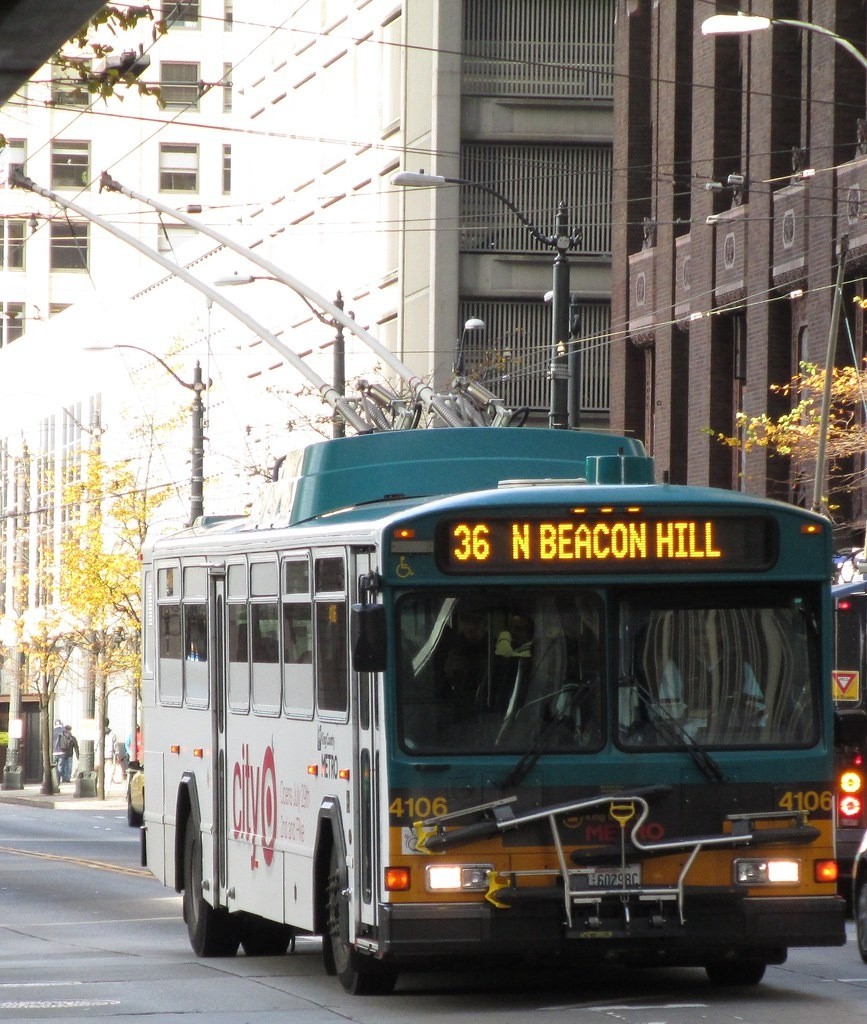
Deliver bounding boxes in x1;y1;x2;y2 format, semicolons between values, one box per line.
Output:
77;339;216;530
387;162;587;429
212;269;357;439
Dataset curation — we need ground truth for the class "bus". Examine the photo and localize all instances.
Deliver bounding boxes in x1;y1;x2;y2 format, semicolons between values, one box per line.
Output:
6;161;866;998
